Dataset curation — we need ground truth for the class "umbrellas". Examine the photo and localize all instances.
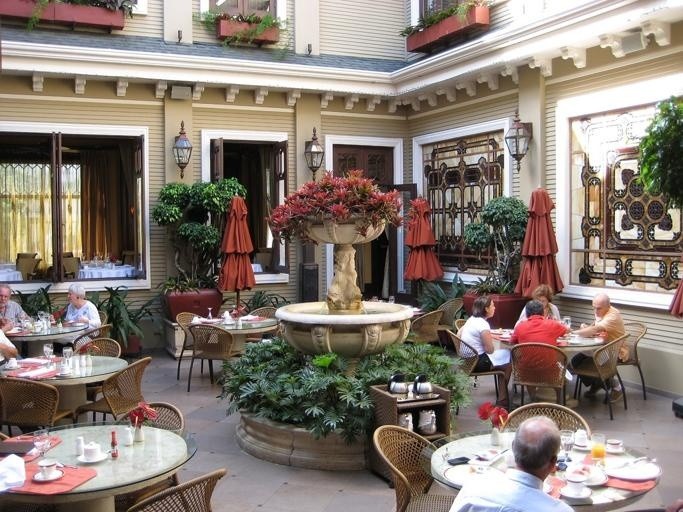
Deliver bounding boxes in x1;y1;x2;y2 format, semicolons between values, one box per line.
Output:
667;280;683;317
403;195;445;296
216;196;256;317
514;188;564;298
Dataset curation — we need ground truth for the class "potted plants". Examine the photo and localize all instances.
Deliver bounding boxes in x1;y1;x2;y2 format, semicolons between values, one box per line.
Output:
264;168;419;247
397;1;491;52
203;9;281;52
0;0;139;35
463;196;530;329
154;177;248;321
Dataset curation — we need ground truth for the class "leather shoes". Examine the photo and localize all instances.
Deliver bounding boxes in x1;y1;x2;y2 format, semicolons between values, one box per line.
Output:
584;385;602;398
608;387;625;403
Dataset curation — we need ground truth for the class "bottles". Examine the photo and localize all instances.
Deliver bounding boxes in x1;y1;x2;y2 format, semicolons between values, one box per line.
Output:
73;366;92;377
73;354;92;369
76;422;144;458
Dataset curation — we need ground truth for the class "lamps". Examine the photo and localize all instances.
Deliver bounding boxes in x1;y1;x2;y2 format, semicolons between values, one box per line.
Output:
305;126;324;182
504;108;531;172
172;120;193;179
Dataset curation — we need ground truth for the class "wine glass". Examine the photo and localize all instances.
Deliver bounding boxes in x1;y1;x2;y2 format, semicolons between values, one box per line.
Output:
563;316;571;328
43;344;73;370
33;430;50;459
560;430;575;462
205;307;215;323
369;296;396;305
17;311;51;333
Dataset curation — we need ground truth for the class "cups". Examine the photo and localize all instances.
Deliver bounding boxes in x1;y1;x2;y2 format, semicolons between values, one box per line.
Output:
38;444;101;479
60;366;69;375
574;429;623;459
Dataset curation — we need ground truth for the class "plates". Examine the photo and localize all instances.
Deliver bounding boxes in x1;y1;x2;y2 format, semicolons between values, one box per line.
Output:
3;365;21;370
441;446;663;500
32;452;109;482
556;331;600;344
55;373;72;377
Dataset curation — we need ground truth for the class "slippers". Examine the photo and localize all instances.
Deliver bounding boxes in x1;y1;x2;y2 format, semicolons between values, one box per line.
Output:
495;398;519;410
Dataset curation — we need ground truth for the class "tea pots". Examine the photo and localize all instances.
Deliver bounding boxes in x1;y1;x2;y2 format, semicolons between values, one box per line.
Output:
387;372;438;435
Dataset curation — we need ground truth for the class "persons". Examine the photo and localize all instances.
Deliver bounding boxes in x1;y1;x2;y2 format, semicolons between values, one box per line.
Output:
516;284;561;323
0;284;27;327
0;328;18;368
509;299;571;406
571;293;625;403
455;296;519;410
52;282;101;357
445;415;575;512
